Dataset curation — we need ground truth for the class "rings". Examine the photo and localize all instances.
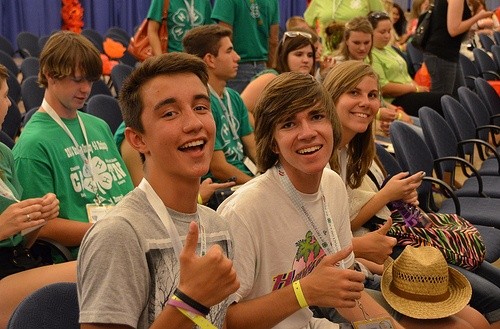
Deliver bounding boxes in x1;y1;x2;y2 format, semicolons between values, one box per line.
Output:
26;214;31;222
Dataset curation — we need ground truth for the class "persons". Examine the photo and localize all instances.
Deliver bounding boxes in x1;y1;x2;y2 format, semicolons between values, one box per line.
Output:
12;31;135;265
239;0;500;212
77;52;240;329
216;70;405;329
0;65;60;282
182;25;258;185
113;121;237;206
147;0;213;56
210;0;281;94
349;141;500;329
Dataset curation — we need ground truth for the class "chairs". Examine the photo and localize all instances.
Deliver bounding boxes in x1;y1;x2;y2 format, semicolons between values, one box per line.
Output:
0;30;500;328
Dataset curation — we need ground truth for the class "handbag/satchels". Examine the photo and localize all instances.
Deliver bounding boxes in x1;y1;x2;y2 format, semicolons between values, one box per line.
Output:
376;198;486;271
128;0;169;61
411;4;433;48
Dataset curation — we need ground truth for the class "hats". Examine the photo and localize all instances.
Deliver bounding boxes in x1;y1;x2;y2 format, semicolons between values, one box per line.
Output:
380;245;472;319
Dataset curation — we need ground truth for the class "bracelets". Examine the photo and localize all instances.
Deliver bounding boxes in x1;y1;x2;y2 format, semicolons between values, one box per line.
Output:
168;288;212;325
415;86;420;92
397;113;401;121
292;280;308;307
375;109;381;121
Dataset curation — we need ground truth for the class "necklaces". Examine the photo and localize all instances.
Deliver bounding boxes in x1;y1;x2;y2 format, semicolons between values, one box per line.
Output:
206;83;259;176
184;0;199;30
249;0;260;19
41;98;112;223
332;0;343;24
0;179;47;236
138;177;207;326
373;46;406;77
322;60;491;329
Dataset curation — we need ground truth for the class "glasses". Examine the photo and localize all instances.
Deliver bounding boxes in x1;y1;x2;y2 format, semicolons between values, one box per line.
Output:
372;12;386;24
283;30;313;44
